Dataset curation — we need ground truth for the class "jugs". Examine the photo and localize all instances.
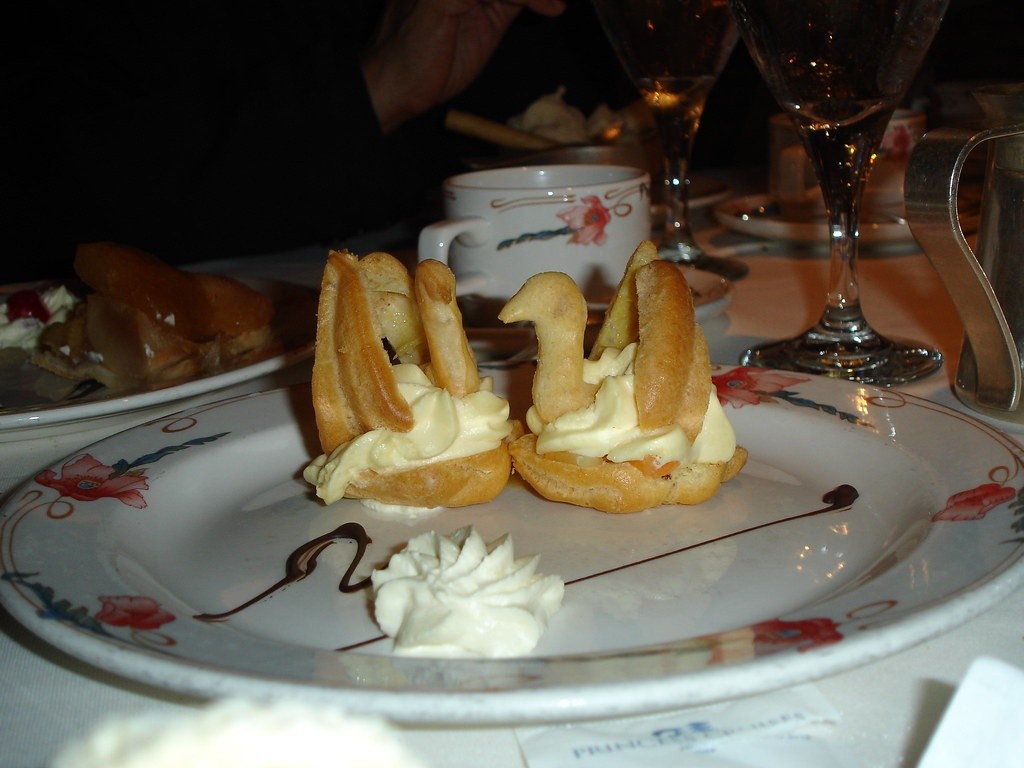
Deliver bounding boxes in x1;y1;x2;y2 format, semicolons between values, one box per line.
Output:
903;82;1024;426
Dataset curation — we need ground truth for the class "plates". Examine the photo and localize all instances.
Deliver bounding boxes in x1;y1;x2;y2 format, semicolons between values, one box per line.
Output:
0;357;1024;728
0;257;323;443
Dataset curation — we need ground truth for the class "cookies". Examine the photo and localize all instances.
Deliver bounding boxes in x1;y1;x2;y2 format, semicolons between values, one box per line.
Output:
496;241;749;514
315;248;521;506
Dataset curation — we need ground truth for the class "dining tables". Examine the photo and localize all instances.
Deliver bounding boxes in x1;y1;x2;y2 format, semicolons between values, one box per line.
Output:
0;140;1024;768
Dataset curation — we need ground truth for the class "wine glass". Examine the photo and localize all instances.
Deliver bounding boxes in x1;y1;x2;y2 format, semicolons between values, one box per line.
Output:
736;0;944;388
589;0;749;281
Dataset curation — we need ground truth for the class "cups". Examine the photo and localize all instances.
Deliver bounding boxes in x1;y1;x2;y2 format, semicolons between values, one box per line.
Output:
858;109;927;223
416;162;652;326
768;112;827;223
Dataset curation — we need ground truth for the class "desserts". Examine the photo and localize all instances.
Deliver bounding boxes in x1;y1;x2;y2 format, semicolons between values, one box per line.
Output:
0;243;315;402
369;524;567;656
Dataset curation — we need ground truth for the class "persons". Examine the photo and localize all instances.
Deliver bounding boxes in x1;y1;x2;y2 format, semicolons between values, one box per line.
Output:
0;0;566;284
